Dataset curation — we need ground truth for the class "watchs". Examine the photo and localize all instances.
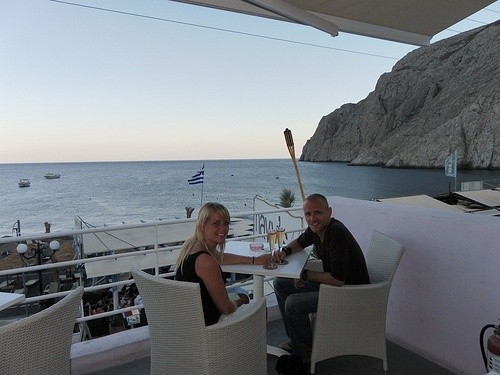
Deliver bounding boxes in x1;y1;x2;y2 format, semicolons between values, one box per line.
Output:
301;268;309;283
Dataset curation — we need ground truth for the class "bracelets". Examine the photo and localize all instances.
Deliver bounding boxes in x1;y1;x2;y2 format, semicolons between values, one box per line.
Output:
248;256;251;265
282;246;292;256
252;256;254;265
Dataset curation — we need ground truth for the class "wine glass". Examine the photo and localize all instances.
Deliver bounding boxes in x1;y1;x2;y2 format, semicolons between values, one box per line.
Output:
267;229;277;269
276;228;288;265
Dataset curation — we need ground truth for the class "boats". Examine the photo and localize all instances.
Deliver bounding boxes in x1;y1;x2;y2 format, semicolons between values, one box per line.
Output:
43;172;62;179
18;178;32;187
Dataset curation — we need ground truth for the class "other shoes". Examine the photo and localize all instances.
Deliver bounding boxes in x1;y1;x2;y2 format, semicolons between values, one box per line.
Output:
278;342;292;351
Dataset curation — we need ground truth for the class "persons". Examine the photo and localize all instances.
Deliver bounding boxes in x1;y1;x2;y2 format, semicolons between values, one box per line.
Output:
82;283;137;316
273;194;370;362
173;203;274;327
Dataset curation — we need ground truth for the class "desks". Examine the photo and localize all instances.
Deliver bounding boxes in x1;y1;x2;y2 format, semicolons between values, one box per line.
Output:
219;241;314;357
0;292;25;312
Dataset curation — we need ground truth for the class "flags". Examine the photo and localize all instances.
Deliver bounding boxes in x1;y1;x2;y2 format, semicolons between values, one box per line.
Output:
188;170;204;185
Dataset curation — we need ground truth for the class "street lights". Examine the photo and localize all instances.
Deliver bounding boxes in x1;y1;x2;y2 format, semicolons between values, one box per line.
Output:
17;239;60;312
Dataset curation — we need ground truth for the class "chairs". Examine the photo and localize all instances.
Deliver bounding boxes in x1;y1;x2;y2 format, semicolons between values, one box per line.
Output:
129;269;267;375
0;286;84;375
303;230;405;375
